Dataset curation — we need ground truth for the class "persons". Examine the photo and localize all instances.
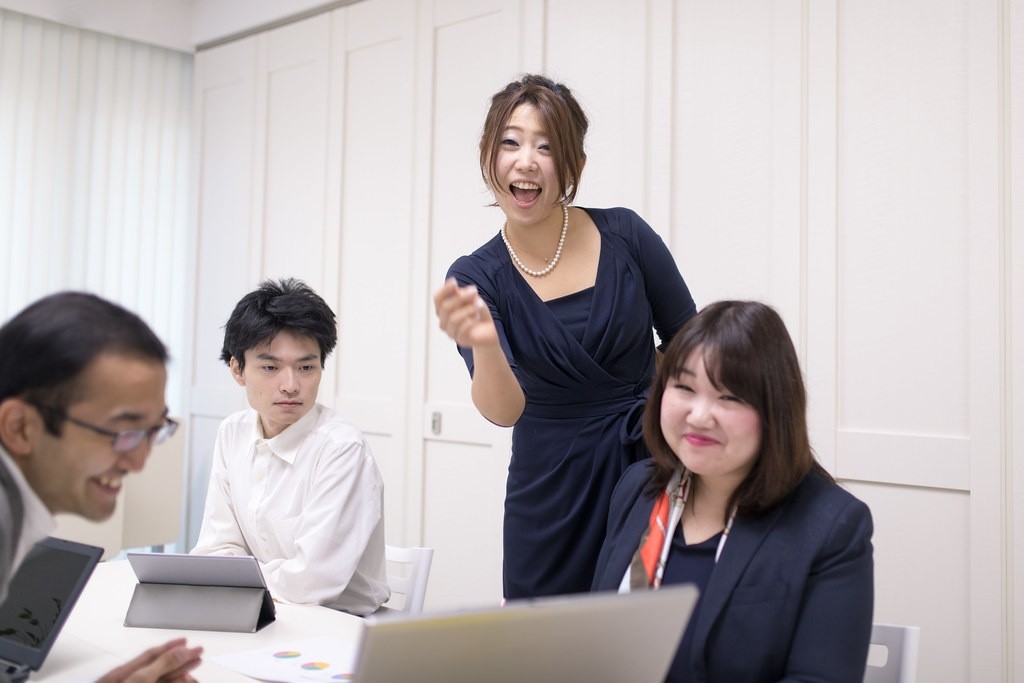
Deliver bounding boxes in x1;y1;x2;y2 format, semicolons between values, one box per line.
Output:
188;278;392;619
587;301;876;683
434;75;696;605
0;290;204;683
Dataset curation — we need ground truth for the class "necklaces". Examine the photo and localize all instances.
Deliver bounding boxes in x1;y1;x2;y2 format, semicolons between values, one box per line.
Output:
500;203;569;277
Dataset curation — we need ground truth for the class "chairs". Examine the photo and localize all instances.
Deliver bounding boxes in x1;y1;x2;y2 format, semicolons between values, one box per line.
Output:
377;543;433;617
860;622;920;683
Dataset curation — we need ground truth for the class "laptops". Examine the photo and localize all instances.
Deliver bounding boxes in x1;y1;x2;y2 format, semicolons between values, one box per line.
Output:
351;584;699;683
0;535;105;683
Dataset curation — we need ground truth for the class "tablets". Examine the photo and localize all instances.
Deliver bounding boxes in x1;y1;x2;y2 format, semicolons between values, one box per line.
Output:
128;552;276;618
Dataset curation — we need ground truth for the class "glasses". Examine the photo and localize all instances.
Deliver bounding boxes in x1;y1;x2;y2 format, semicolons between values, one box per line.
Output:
63;410;180;452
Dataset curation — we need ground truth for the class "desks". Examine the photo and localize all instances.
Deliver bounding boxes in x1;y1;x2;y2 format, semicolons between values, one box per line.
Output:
28;555;369;683
